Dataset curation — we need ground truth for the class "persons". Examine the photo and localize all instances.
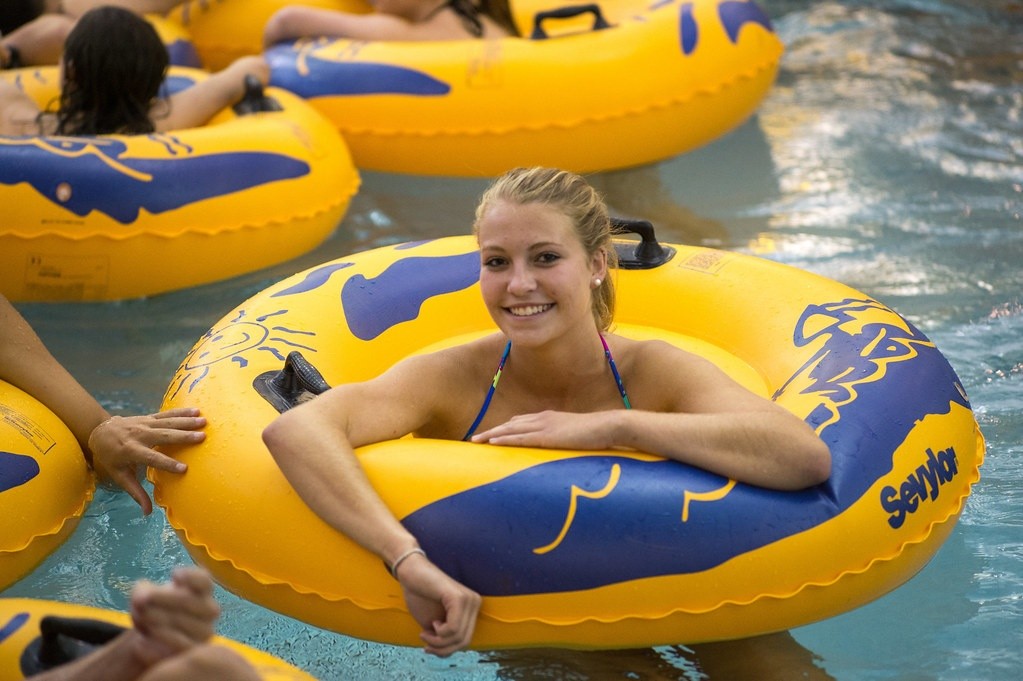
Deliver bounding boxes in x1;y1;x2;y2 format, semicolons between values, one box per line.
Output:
258;164;861;681
0;0;81;68
62;1;182;28
0;282;208;517
0;6;269;144
264;0;522;49
16;565;272;681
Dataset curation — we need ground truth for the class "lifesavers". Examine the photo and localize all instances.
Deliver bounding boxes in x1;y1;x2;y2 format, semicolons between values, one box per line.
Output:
2;378;93;591
2;0;787;303
0;599;320;681
153;230;986;649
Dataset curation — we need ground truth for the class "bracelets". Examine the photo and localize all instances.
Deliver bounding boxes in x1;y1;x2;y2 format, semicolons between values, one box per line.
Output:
386;548;427;581
